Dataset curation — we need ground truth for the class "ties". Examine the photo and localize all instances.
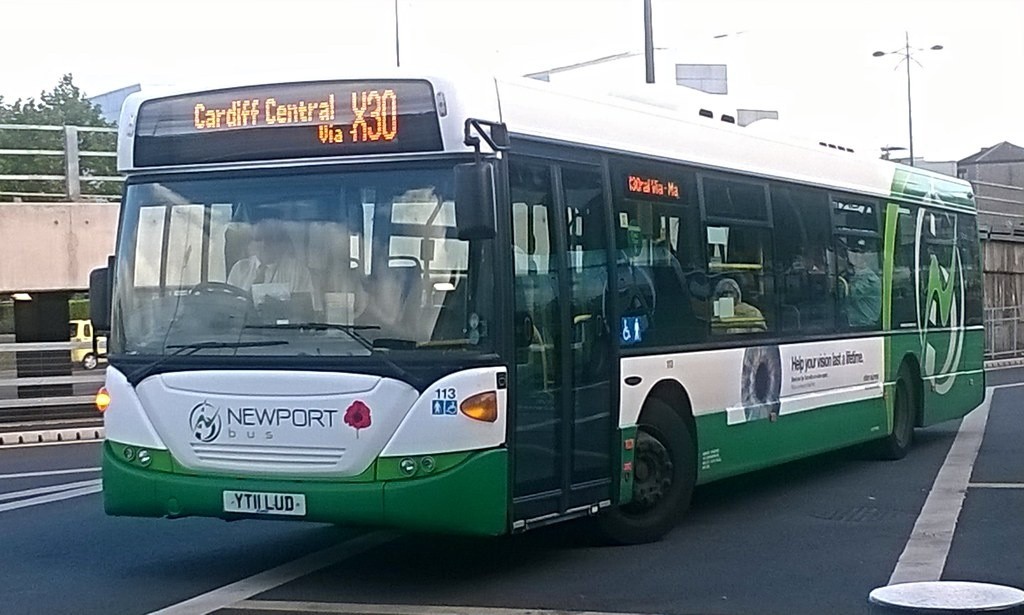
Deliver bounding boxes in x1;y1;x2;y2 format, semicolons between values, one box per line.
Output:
251;264;268;284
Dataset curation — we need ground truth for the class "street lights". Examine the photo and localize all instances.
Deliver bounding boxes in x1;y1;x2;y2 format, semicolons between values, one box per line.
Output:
872;29;944;168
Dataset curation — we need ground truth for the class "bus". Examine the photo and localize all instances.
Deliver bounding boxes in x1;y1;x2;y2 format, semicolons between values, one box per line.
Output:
89;72;986;548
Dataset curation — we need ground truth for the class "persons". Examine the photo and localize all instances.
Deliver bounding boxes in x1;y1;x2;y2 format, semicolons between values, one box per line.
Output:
710;279;768;333
832;246;883;330
227;217;316;308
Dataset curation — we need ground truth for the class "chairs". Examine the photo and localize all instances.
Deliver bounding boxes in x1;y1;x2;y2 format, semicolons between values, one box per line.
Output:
318;255;833;386
